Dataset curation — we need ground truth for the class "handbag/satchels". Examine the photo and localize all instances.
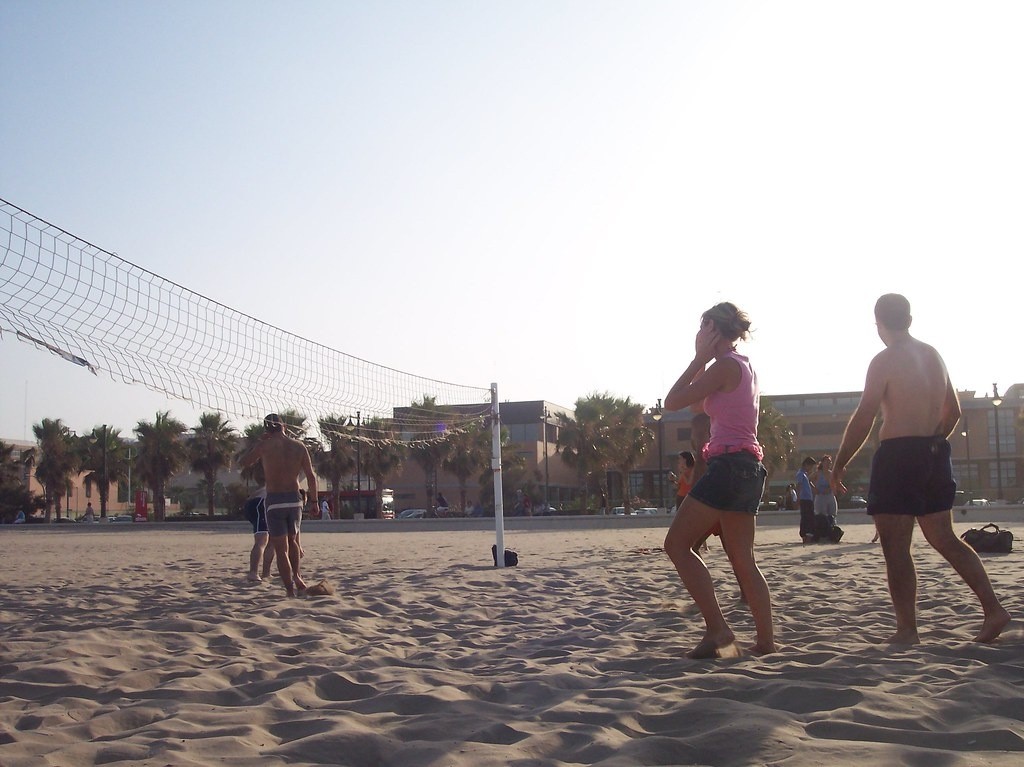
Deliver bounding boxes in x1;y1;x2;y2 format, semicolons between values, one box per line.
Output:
832;525;844;543
960;524;1013;553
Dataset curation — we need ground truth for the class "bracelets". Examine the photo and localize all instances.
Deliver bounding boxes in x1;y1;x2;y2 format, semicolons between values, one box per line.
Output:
312;500;318;503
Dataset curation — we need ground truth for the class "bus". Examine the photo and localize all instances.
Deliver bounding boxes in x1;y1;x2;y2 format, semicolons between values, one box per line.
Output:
301;489;396;519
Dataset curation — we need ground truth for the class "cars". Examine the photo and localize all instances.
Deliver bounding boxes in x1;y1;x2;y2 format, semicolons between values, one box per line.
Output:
849;495;867;508
53;514;133;523
962;498;991;506
397;508;428;519
635;508;657;517
610;506;638;516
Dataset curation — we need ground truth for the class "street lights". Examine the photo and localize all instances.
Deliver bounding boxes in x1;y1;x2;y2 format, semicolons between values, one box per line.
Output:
991;381;1004;499
960;415;974;506
88;424;109;518
651;398;665;509
538;405;553;513
116;448;132;506
345;410;365;514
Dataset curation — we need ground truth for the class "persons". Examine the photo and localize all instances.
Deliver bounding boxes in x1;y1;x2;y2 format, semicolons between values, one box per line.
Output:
246;434;275;582
463;499;475;518
79;501;95;523
810;455;847;526
668;450;709;551
687;413;748;613
515;488;532;517
322;496;332;520
599;488;610;515
797;456;816;545
830;293;1012;645
242;413;320;598
785;483;798;504
434;492;450;518
14;508;25;524
665;303;780;659
